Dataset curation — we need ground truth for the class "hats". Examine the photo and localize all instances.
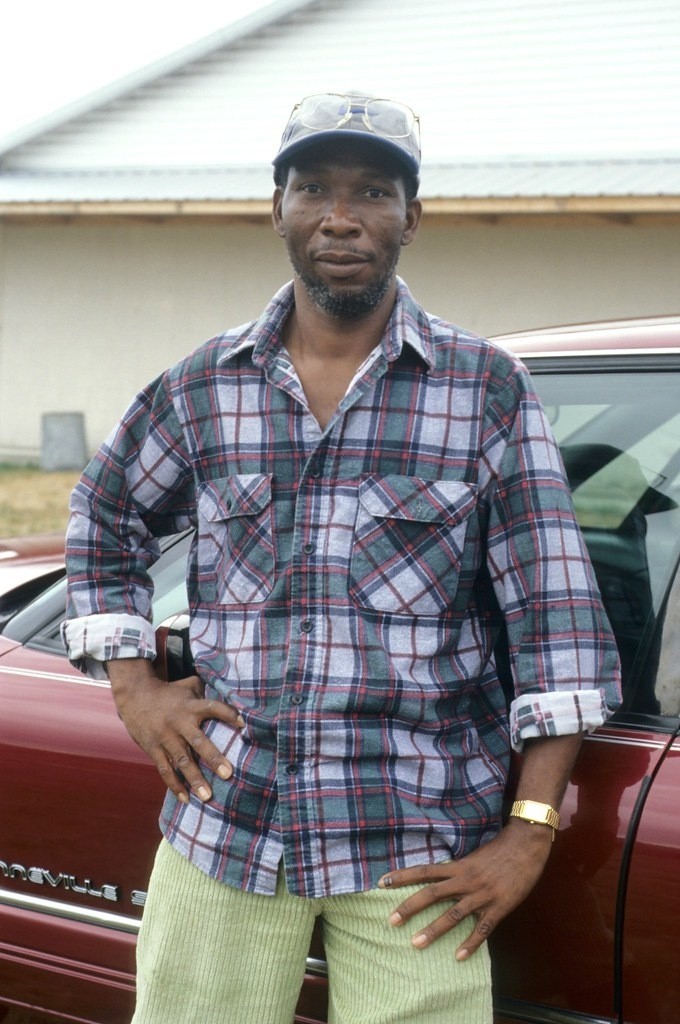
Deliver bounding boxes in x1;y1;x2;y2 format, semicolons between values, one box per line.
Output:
273;89;420;200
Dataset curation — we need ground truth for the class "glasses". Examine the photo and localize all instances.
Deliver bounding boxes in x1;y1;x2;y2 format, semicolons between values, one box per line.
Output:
281;93;421;160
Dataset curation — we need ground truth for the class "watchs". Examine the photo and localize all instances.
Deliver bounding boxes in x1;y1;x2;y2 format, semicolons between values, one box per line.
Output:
510;800;561;831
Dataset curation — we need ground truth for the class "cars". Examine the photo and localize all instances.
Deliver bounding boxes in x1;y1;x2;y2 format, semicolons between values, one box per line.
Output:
0;314;680;1023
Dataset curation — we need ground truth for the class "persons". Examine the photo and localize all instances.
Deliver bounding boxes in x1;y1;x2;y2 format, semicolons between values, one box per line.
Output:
60;91;624;1024
